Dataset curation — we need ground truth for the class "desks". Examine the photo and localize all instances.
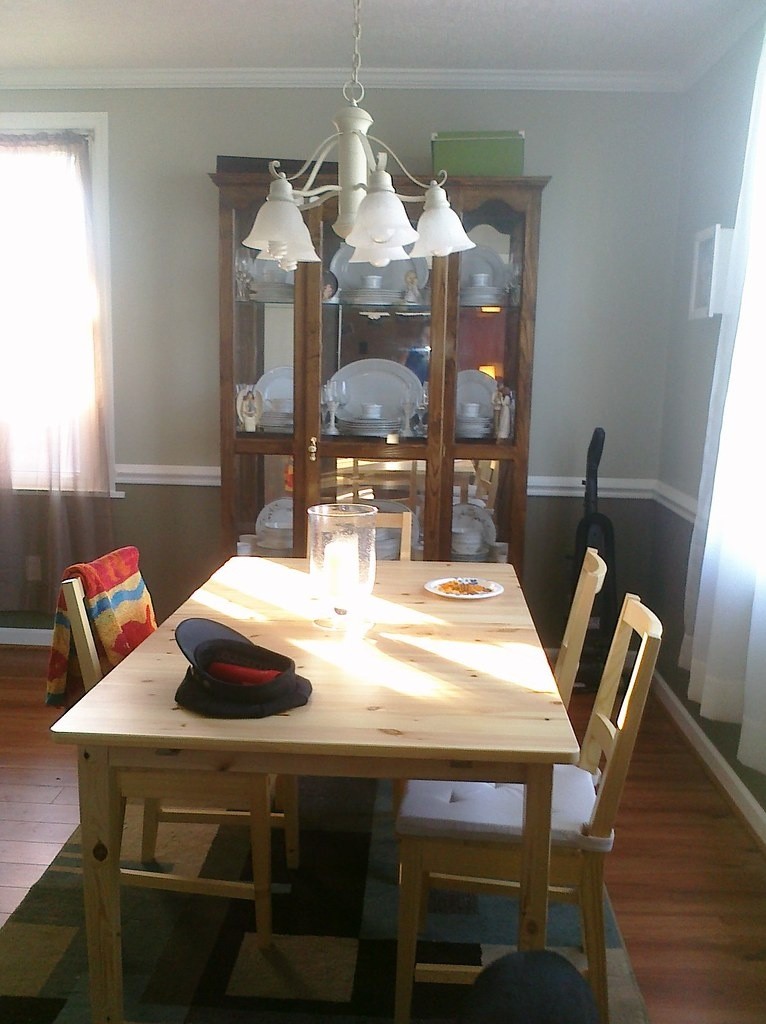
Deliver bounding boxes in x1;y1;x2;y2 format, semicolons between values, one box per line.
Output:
358;460;477;505
51;556;580;1024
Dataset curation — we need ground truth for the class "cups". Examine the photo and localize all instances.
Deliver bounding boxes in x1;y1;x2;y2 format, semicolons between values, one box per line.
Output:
262;269;286;283
361;402;382;419
236;542;252;556
470;272;490;287
492;541;509;563
359;275;382;289
264;397;293;411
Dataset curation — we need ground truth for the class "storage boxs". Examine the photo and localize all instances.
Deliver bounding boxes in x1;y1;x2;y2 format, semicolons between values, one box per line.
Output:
430;130;525;175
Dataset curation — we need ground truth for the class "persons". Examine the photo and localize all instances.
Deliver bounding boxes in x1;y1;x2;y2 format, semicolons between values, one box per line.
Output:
406;320;431;383
492;381;516;439
243;391;257;432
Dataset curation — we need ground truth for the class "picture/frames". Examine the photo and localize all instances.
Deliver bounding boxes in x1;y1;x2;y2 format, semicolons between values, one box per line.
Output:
687;223;721;320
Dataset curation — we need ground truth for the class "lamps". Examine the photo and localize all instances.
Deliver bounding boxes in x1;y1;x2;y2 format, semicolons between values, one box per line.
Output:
241;1;477;273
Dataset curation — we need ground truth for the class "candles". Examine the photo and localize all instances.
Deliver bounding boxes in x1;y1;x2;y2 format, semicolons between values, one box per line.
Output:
323;532;359;588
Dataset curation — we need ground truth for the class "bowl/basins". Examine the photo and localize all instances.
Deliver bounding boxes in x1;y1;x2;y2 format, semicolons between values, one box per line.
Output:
261;521;293;538
451;527;483;553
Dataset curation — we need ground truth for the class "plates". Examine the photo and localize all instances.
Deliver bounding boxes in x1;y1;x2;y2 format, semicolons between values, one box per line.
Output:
447;504;498;560
252;254;297;303
424;578;505;599
329;242;430;306
456;244;513;308
255;499;302;552
359;497;419;560
255;366;299;434
327;358;424;436
447;371;501;445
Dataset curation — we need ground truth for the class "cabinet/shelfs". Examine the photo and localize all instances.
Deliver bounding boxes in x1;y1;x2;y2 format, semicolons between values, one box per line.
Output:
208;171;553;584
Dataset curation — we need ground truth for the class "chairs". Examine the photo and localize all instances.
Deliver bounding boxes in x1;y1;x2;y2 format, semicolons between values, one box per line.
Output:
45;546;300;952
306;511;413;562
352;459;417;516
554;547;608;715
474;459;499;513
394;593;664;1024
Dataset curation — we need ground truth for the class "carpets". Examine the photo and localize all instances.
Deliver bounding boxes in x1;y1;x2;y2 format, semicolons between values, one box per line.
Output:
0;798;652;1024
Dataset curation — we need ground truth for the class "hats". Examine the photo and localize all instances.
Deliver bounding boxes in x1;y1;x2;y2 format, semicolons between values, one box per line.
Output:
176;617;312;720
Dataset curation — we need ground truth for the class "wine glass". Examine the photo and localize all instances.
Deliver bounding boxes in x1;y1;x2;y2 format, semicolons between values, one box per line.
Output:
396;381;429;438
326;380;349;420
